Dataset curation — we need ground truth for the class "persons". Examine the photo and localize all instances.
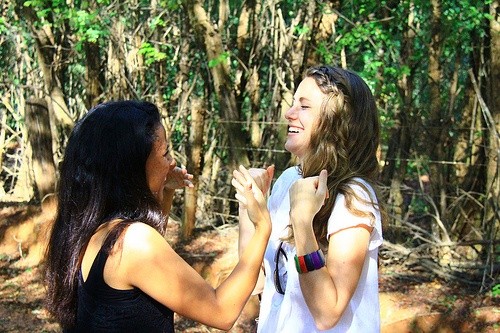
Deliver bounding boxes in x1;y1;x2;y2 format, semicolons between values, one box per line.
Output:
231;62;383;333
37;99;273;332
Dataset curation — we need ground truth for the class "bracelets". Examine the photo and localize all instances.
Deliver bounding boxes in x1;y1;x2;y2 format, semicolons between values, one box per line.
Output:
293;248;326;274
